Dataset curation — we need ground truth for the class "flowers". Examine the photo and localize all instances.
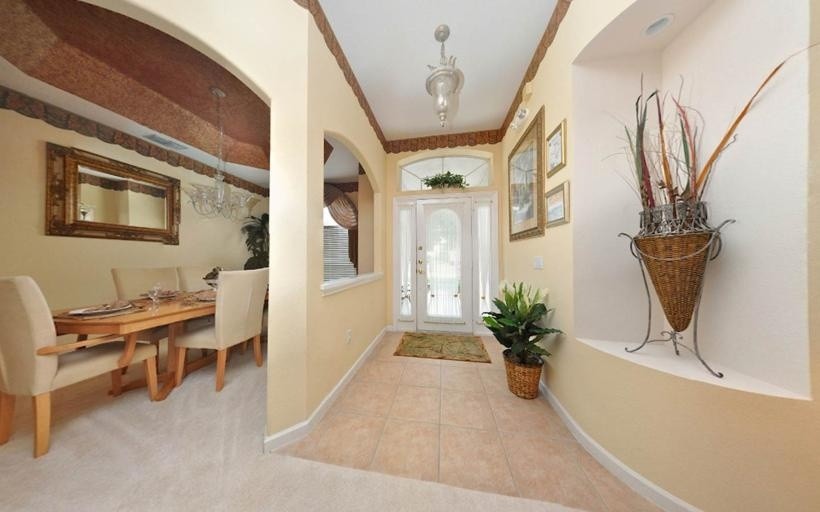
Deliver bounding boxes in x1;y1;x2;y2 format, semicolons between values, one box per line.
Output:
482;279;566;361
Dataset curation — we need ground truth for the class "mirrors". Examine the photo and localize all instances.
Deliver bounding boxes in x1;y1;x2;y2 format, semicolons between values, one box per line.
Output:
45;141;180;246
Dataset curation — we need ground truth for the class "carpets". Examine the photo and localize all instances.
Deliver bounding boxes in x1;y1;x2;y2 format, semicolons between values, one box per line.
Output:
393;330;492;363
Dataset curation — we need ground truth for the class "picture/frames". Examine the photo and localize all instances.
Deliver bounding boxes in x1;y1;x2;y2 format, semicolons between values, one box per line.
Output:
507;103;571;242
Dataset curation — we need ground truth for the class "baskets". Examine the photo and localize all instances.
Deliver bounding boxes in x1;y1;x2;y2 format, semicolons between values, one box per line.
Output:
637;234;717;332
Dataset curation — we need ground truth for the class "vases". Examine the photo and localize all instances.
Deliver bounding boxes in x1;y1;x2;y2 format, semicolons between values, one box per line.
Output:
502;348;544;400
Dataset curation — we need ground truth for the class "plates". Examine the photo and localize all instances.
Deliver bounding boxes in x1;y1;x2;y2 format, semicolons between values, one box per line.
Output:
68;301;133;317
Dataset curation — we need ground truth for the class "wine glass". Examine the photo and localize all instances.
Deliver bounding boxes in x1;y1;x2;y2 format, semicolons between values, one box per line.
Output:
145;281;165;316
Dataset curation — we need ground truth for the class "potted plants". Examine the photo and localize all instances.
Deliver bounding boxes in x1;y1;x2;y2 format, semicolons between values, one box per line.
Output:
420;171;470;191
602;43;819;378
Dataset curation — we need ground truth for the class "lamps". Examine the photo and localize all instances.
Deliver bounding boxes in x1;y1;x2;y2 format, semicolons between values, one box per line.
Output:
425;25;462;129
180;86;262;224
